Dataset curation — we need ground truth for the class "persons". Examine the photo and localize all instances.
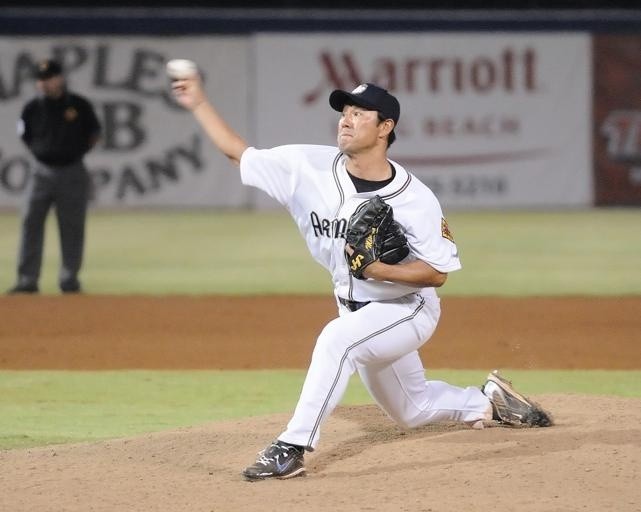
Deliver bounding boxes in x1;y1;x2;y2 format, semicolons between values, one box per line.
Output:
165;55;556;482
2;57;104;296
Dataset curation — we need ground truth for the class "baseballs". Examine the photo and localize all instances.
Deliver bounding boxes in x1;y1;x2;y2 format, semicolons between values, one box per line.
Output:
167;58;197;79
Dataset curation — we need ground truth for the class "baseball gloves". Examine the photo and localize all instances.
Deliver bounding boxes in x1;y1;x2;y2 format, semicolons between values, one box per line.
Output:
343;197;410;280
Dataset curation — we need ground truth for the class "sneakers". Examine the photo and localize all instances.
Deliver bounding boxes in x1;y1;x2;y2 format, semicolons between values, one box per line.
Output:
242;441;307;481
483;370;551;428
61;276;79;291
8;282;36;293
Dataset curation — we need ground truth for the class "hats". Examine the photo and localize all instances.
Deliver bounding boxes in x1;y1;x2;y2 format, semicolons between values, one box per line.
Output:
34;61;62;79
329;84;400;127
